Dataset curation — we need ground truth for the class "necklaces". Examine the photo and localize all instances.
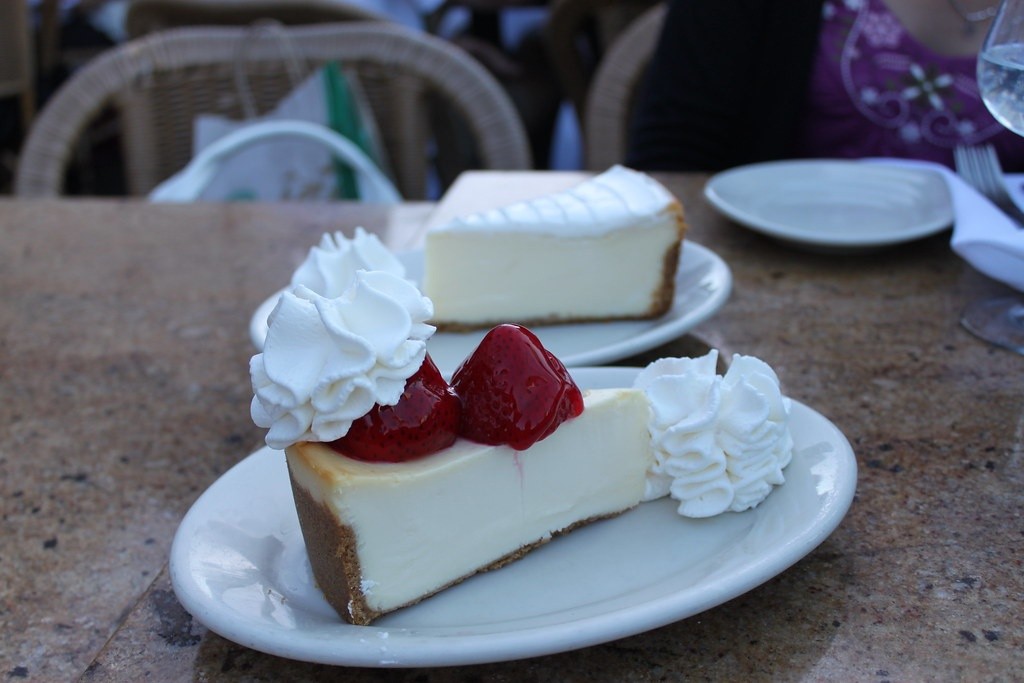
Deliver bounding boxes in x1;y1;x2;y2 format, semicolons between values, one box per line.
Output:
950;0;997;35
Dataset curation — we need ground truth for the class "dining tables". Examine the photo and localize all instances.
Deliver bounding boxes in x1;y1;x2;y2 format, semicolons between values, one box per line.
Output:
0;183;1024;683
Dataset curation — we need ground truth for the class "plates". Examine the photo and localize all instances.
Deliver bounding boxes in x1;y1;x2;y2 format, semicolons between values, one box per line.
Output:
167;370;857;668
247;241;733;372
703;160;952;246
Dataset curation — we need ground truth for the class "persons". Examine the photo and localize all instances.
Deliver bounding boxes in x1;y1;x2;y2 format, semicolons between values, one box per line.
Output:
625;0;1024;173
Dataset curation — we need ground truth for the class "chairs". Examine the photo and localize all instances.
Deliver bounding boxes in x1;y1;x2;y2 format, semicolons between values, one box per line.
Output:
15;24;532;204
587;10;668;175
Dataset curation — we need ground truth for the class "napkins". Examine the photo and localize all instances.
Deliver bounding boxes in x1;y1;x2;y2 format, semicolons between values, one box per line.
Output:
946;172;1024;287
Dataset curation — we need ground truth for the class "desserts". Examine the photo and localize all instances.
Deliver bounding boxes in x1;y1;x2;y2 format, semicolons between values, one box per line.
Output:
248;225;796;627
419;163;688;332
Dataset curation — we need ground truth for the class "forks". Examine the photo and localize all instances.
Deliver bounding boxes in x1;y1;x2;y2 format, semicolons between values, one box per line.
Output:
952;145;1024;229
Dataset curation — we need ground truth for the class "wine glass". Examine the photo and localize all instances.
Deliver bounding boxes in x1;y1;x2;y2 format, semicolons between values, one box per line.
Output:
960;0;1024;353
